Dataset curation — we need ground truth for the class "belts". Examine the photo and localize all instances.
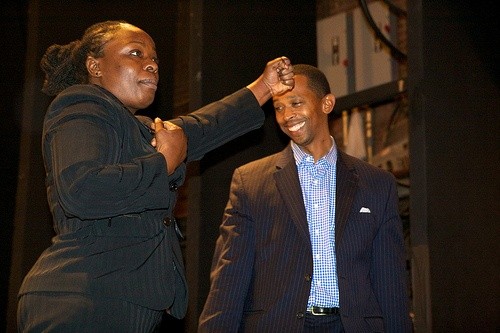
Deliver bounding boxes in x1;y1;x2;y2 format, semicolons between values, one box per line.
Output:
307;306;340;316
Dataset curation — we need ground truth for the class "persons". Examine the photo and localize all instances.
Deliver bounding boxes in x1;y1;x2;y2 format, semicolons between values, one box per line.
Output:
195;65;414;333
14;21;295;333
